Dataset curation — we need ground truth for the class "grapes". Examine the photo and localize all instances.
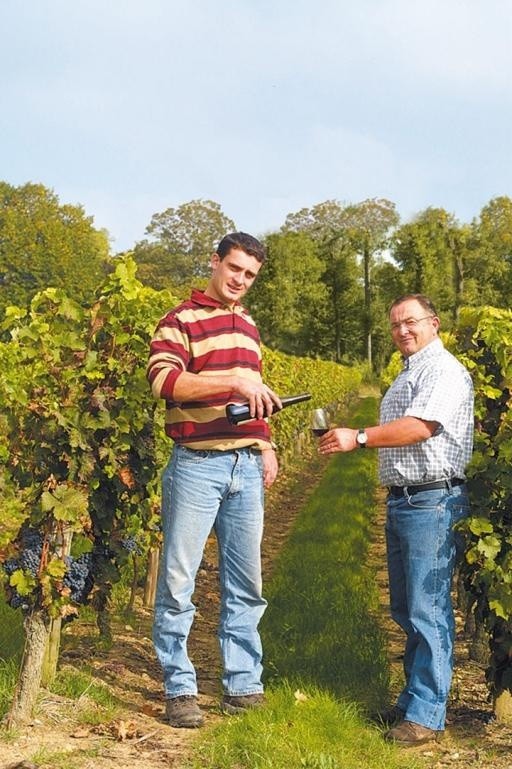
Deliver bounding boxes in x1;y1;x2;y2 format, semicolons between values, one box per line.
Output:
0;434;162;623
450;350;512;726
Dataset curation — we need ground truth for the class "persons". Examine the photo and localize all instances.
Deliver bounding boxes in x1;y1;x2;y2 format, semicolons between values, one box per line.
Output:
147;232;278;727
317;294;474;749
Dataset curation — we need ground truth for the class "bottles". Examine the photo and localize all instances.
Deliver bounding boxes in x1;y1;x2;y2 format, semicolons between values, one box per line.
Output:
225;393;312;427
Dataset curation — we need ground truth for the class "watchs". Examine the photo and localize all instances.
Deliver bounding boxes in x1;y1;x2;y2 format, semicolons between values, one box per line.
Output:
357;428;367;448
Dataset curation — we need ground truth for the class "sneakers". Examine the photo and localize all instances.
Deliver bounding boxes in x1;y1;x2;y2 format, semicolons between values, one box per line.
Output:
384;721;444;747
222;693;267;715
372;706;405;725
166;694;204;728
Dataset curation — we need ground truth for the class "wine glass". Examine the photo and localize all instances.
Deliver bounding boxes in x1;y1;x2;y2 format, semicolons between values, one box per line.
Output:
309;408;330;455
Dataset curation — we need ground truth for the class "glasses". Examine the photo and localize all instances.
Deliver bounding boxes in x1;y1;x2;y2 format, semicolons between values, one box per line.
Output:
389;316;431;332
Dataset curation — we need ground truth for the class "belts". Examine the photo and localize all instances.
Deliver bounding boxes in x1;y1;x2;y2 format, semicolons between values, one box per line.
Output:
387;478;463;496
